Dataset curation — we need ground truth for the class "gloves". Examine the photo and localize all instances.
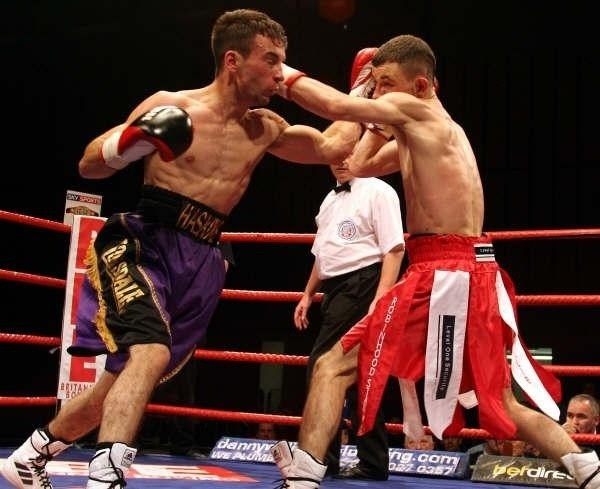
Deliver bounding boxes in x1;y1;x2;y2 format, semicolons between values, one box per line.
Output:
365;76;438;142
100;105;195;171
350;48;379;99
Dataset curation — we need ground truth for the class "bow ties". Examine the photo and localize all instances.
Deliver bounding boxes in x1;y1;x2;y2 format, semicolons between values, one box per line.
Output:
332;182;351;193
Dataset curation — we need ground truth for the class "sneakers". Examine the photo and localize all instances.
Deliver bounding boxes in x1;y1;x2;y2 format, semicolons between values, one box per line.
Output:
559;447;600;488
83;442;137;489
270;439;330;489
0;426;74;489
334;461;391;481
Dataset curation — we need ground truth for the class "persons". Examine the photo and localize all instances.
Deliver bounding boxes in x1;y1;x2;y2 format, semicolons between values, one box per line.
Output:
294;147;406;481
339;427;349;445
387;394;600;468
254;421;276;439
267;34;599;489
1;11;380;489
